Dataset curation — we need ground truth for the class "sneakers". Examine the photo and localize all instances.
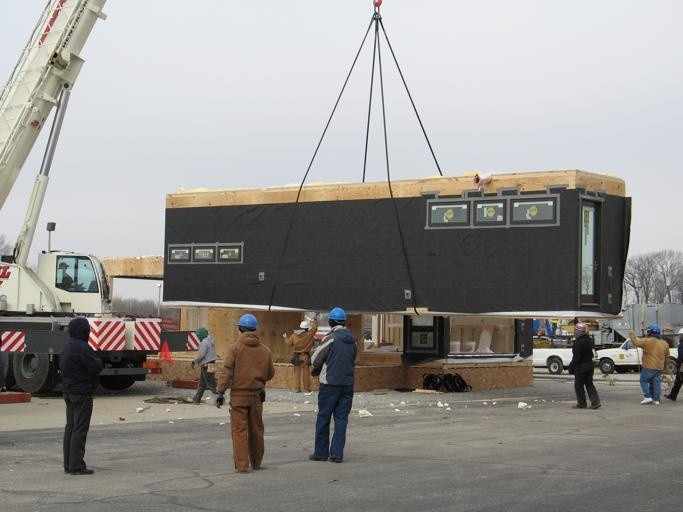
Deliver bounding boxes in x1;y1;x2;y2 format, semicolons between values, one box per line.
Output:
330;456;342;463
572;404;587;409
309;454;328;461
654;400;659;405
590;403;601;409
641;397;652;404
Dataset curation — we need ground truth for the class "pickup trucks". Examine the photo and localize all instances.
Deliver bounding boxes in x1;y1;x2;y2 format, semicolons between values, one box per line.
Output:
595;332;683;374
531;348;574;374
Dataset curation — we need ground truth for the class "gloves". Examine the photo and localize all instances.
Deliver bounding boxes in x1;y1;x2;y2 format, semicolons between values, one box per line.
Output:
216;394;223;408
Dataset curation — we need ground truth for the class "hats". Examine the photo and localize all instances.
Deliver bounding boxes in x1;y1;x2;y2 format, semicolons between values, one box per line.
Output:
59;262;69;267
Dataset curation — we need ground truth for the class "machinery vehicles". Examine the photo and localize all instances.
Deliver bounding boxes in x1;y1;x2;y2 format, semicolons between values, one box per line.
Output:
0;0;165;398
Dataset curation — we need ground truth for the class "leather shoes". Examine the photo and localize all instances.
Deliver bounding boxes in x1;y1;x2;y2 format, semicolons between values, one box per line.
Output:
664;394;676;401
64;468;93;475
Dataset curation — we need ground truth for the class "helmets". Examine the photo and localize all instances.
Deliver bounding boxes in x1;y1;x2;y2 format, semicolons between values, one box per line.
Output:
328;307;346;321
300;321;309;329
234;313;257;328
573;323;587;334
646;324;660;336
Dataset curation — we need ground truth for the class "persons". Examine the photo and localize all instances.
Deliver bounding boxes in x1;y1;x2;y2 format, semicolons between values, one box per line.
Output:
566;322;601;410
280;313;319;394
308;307;358;462
55;261;78;290
627;323;683;406
212;313;274;474
185;326;217;404
56;315;102;475
